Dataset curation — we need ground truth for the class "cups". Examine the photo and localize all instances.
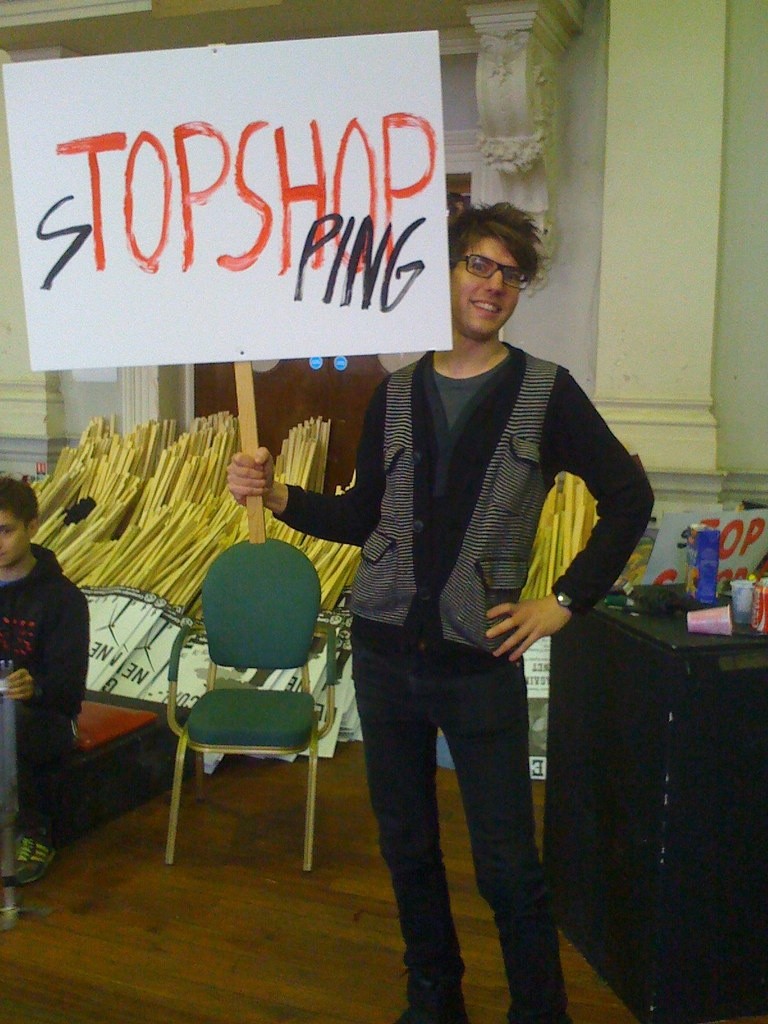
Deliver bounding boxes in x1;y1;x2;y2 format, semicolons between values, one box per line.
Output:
686;603;734;636
729;580;755;624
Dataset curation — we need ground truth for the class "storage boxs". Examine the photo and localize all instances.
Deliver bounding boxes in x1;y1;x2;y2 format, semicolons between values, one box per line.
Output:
685;522;719;603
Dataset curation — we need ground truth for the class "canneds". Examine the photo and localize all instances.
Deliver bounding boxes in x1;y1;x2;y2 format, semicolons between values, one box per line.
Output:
749;577;768;633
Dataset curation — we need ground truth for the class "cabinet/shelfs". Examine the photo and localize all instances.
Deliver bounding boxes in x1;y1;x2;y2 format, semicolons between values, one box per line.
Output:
543;581;768;1024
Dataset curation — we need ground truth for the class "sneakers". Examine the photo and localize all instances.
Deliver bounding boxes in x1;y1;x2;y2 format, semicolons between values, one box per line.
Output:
13;831;59;887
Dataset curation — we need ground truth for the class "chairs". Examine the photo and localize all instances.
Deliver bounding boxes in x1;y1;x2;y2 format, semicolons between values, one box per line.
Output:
166;538;338;871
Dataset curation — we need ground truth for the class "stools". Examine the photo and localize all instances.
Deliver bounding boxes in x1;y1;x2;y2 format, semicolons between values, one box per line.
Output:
62;688;194;836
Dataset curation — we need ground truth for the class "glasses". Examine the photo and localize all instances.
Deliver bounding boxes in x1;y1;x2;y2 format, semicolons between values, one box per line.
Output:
450;250;534;294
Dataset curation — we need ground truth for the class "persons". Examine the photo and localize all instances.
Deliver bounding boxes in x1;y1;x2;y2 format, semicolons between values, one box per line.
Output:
0;476;91;889
228;199;656;1024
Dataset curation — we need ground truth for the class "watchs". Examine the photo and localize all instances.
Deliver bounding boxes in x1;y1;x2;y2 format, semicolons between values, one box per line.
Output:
549;584;584;617
